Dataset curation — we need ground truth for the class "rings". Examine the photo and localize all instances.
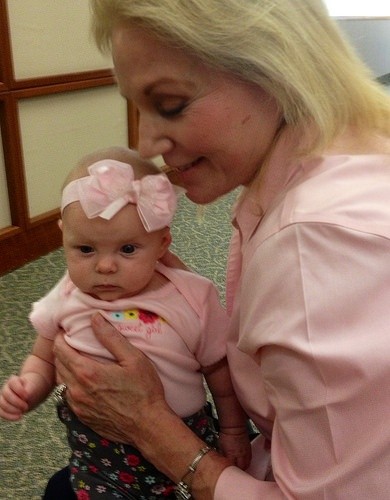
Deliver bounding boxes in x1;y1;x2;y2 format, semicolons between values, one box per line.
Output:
51;384;68;407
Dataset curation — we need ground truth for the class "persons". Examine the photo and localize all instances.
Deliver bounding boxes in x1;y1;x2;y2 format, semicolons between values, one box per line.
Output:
0;148;255;500
49;0;390;500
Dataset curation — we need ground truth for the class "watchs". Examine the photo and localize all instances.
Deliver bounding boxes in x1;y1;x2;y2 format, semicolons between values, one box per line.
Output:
174;444;214;500
